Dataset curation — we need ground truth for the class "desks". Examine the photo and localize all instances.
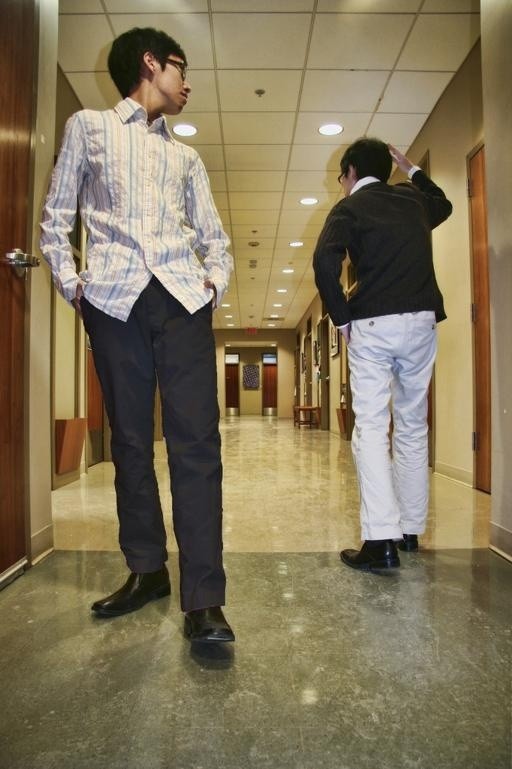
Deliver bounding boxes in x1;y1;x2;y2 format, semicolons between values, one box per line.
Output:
294;406;320;429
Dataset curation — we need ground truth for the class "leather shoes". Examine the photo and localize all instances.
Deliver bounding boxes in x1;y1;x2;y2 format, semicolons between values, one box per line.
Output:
182;604;237;646
340;537;402;574
89;564;174;618
397;532;420;553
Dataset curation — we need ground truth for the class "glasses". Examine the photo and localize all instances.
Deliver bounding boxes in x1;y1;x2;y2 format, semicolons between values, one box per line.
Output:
153;55;187;81
337;162;356;185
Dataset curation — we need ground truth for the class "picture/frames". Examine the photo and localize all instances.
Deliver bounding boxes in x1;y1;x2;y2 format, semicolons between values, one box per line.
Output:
328;315;339;357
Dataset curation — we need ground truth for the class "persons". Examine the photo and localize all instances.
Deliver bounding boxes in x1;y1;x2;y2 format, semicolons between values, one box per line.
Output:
310;139;451;573
42;28;237;648
